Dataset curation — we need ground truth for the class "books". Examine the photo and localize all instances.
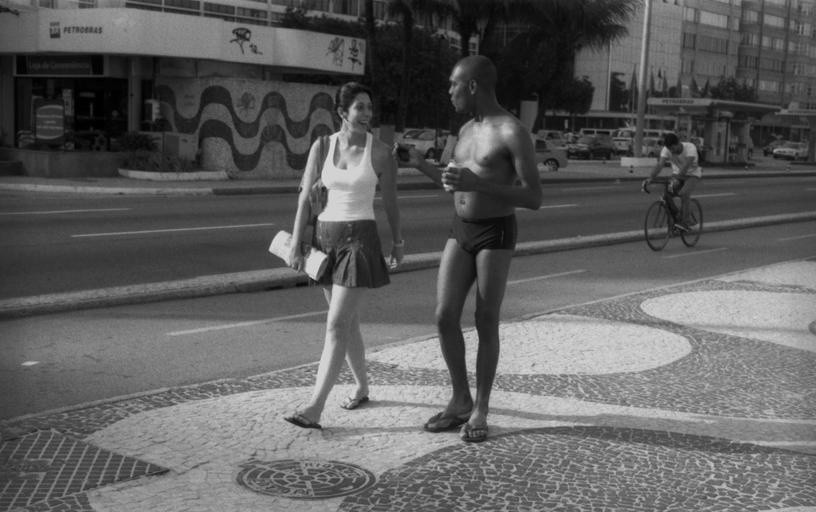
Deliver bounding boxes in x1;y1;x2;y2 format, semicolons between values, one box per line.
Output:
268;230;328;282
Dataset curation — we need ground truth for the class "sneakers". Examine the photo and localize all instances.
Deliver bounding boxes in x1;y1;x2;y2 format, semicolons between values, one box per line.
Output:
674;222;689;233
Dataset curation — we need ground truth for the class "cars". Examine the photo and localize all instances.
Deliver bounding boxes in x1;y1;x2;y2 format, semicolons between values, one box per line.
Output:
567;136;612;160
774;142;809;162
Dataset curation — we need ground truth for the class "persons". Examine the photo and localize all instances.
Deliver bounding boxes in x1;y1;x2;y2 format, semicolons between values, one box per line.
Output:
564;128;573;144
648;134;703;232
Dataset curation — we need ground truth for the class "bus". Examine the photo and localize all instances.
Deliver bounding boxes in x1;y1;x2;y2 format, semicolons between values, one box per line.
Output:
613;128;676;157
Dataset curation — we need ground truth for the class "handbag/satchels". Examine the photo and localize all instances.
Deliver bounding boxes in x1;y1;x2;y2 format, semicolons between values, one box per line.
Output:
308;177;327;224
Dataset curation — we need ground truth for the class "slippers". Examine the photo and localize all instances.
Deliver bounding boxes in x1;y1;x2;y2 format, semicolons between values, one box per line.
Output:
423;408;489;442
283;403;325;432
342;389;370;408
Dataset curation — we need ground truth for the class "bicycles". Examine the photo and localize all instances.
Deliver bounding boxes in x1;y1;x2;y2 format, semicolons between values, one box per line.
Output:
640;179;703;251
